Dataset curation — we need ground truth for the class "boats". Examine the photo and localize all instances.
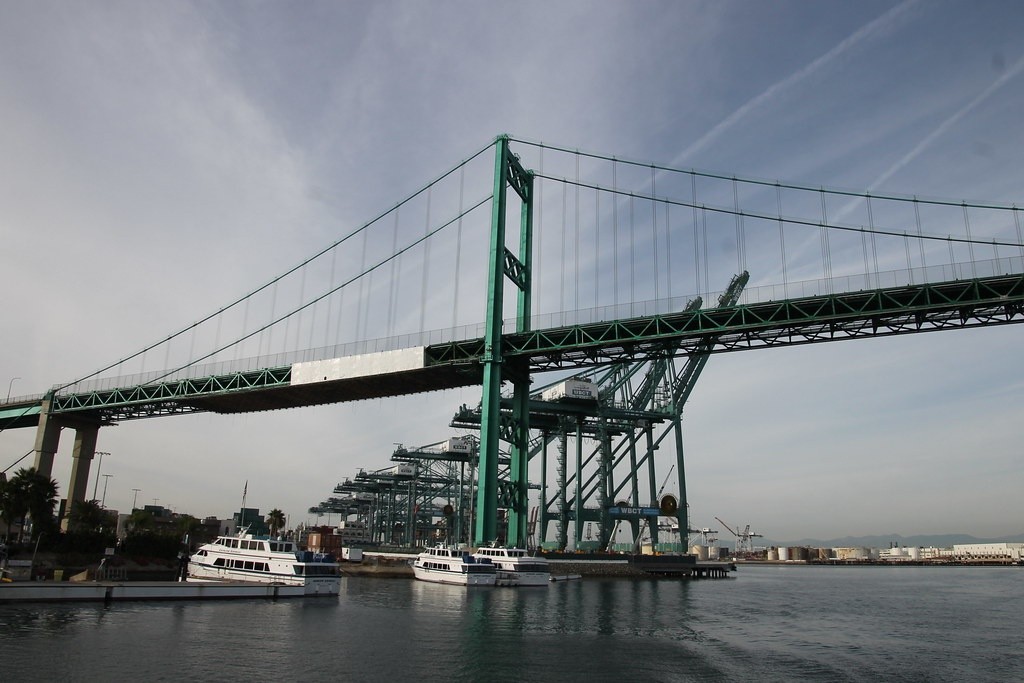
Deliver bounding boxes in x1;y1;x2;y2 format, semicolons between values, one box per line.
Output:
178;478;344;598
471;545;551;586
409;538;497;586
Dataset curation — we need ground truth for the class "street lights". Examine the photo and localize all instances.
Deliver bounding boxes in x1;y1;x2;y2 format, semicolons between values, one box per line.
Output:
131;488;141;510
100;474;113;508
93;451;111;500
153;498;160;506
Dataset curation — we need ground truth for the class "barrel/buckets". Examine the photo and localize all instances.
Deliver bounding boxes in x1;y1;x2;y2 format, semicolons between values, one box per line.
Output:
54;570;63;582
